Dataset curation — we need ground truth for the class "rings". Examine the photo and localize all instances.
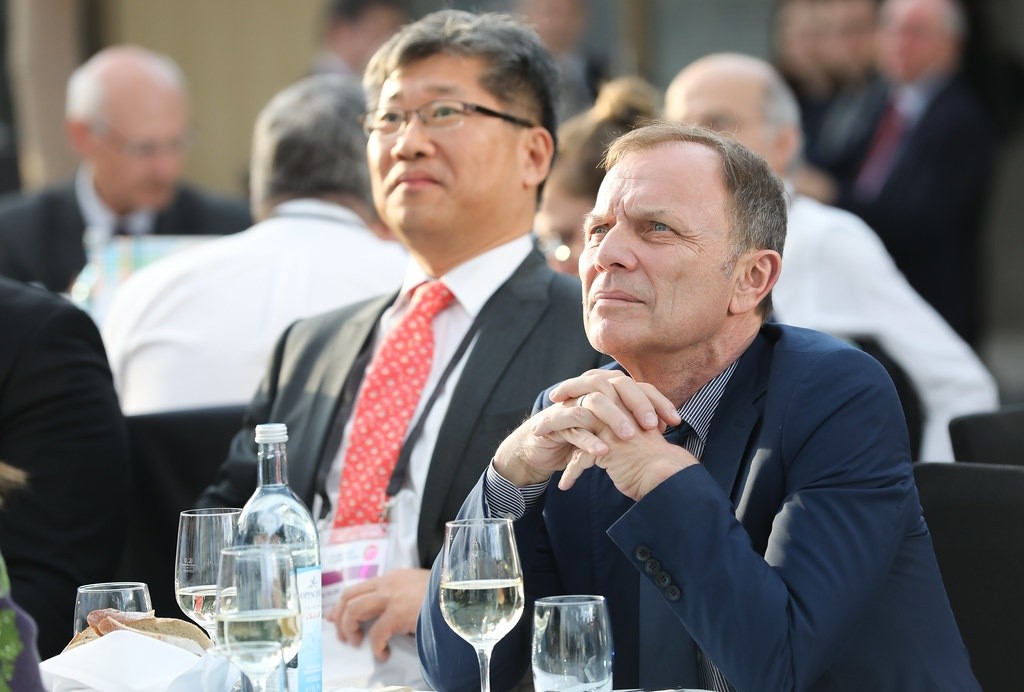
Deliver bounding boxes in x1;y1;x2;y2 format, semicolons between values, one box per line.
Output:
574;394;587;408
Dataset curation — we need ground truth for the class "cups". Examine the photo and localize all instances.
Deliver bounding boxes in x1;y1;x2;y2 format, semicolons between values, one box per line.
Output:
73;582;153;638
212;641;288;692
173;508;243;644
532;595;613;692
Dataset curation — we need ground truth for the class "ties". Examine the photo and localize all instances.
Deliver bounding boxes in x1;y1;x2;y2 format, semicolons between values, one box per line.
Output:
329;280;456;545
846;99;910;204
638;420;702;692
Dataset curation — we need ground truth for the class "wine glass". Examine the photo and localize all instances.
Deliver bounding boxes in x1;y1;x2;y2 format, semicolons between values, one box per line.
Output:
215;543;301;691
439;518;526;692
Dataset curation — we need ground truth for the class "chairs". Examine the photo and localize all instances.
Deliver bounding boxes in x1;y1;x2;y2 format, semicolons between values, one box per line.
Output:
948;406;1024;466
118;404;247;638
904;459;1024;692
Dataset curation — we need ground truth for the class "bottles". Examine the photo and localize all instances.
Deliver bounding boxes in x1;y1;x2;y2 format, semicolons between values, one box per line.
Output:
238;424;322;691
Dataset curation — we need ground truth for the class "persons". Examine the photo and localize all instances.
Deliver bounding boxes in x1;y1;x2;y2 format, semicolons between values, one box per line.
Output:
1;1;1001;691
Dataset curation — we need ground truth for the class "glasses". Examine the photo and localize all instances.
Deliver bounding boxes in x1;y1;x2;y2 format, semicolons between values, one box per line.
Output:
361;98;536;140
